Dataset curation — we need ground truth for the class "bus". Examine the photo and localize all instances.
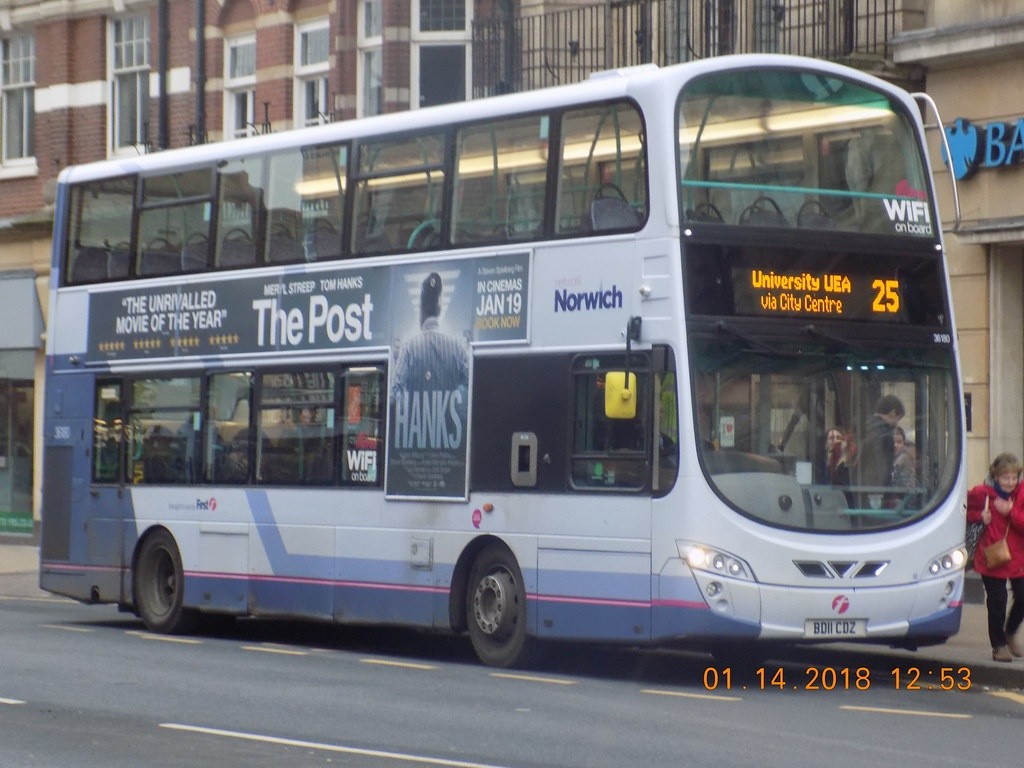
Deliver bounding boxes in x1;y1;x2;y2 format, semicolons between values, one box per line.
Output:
37;51;961;672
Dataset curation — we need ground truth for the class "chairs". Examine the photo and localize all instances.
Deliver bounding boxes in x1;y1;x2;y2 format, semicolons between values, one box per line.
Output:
69;184;919;526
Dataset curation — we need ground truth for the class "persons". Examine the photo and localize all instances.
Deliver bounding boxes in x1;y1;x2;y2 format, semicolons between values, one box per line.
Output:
819;395;919;513
965;452;1024;663
390;272;471;446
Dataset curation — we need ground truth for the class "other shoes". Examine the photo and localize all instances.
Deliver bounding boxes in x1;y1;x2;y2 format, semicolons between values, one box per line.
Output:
992;646;1012;662
1004;634;1022;658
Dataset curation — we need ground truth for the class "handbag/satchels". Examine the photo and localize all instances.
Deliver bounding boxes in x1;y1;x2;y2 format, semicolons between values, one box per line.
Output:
965;495;991;561
985;539;1012;569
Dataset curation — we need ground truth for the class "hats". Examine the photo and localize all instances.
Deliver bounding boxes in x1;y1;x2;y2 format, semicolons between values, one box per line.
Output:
419;271;442;316
